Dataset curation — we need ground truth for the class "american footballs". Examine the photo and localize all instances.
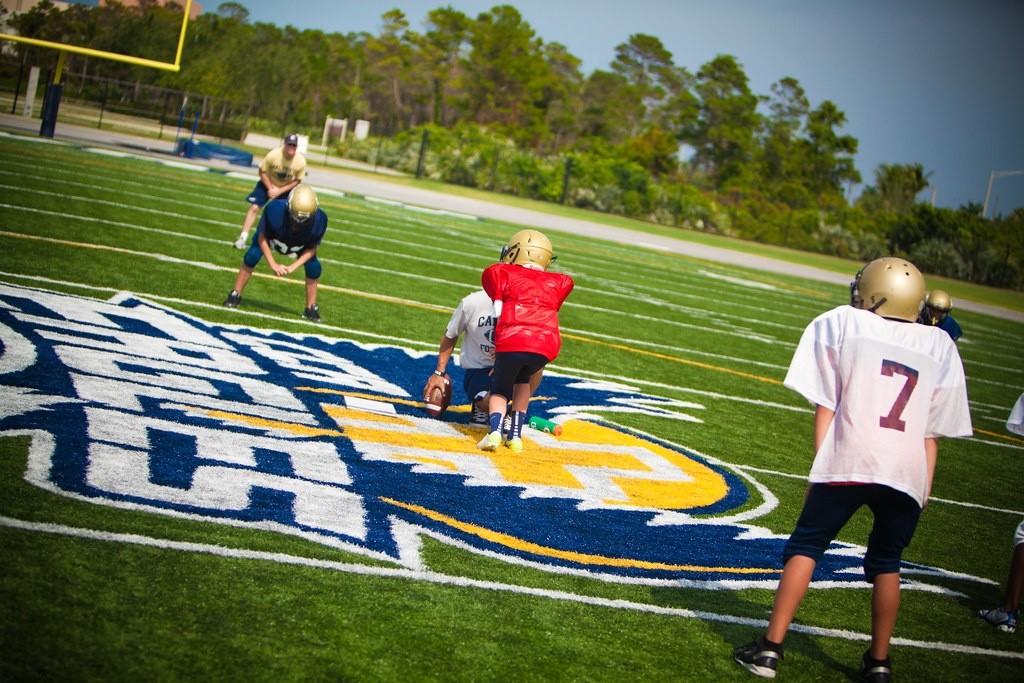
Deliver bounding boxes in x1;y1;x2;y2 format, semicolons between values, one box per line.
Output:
425;373;452;417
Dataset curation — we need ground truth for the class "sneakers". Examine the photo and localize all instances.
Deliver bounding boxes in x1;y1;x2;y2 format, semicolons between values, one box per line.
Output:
978;608;1016;634
505;438;523;453
860;649;891;683
476;431;502;451
733;635;780;678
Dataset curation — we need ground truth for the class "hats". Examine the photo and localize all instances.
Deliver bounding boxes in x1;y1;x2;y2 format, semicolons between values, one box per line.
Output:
284;134;298;145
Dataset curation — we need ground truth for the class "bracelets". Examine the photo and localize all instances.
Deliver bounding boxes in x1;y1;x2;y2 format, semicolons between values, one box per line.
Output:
434;369;445;377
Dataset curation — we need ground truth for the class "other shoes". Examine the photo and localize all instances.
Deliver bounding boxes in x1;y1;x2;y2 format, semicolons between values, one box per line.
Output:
470;400;489;428
224;290;242;308
304;304;321;322
234;236;245;250
504;412;512;434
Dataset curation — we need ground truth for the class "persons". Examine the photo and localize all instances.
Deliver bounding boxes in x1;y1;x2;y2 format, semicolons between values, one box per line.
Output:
733;257;973;683
423;289;544;436
223;184;328;322
915;289;963;343
232;133;307;249
977;393;1023;633
475;228;575;453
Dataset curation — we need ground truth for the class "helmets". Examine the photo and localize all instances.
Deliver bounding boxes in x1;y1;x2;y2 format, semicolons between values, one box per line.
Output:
501;229;552;271
289;184;319;224
926;290;953;313
850;257;927;323
